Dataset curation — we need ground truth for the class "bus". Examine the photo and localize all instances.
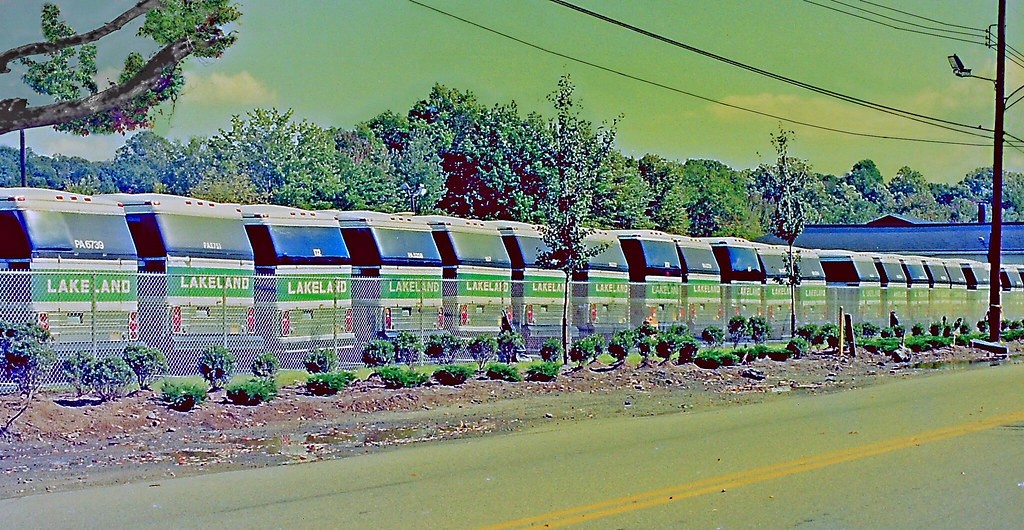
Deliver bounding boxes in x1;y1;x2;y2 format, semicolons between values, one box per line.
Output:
0;187;1024;390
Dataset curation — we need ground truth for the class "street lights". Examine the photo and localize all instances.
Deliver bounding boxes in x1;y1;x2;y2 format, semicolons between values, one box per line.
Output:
947;53;1004;341
